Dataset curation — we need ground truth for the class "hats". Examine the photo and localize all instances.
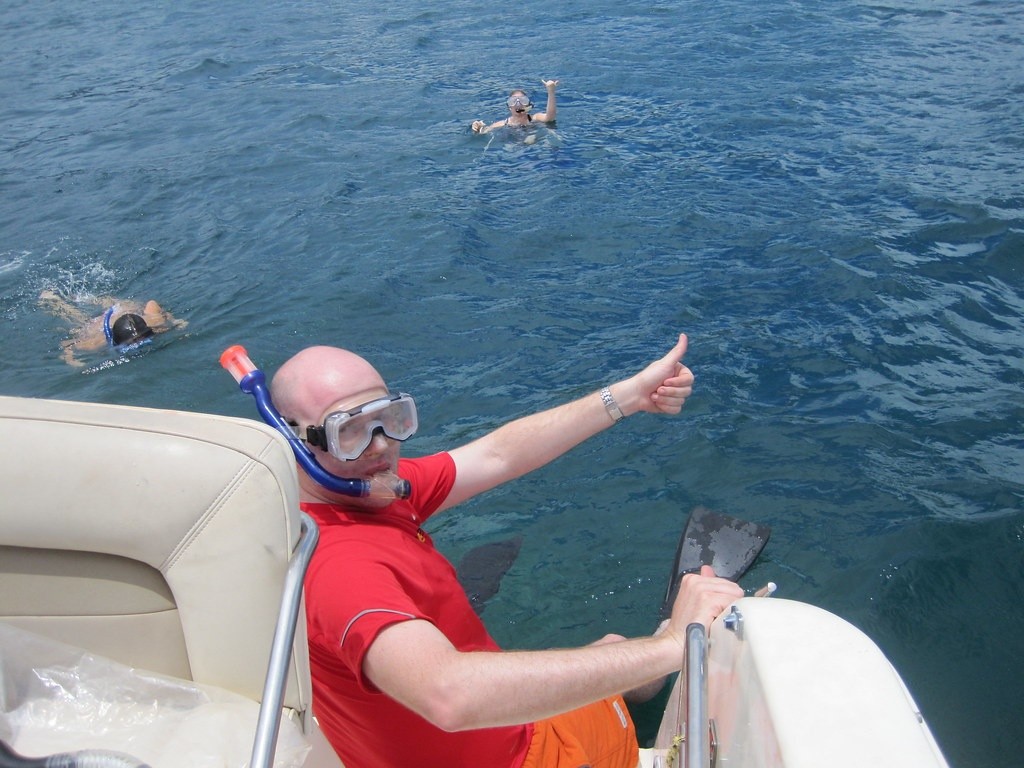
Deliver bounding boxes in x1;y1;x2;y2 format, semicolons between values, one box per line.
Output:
270;347;390;443
111;314;151;346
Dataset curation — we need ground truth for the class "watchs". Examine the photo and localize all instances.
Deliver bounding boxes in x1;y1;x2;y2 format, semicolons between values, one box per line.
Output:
273;333;745;768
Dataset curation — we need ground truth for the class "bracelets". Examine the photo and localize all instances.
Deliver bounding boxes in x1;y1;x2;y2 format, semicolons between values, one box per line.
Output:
601;386;625;423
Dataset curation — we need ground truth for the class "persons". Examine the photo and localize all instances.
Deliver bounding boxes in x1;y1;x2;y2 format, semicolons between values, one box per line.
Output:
472;78;559;136
62;300;187;366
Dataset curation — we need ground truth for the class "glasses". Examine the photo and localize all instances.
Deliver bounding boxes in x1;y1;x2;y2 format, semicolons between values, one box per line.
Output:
292;390;420;461
506;95;530;106
